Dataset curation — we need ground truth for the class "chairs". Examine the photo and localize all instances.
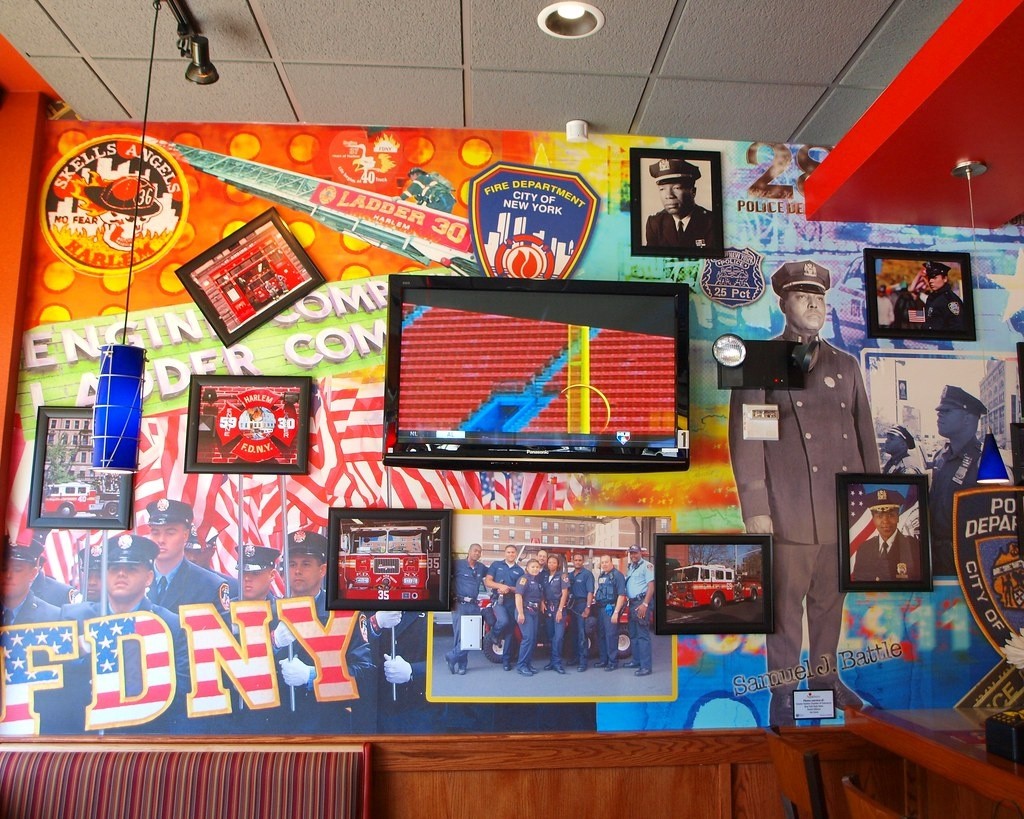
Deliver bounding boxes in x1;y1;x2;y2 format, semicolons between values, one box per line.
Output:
765;733;900;819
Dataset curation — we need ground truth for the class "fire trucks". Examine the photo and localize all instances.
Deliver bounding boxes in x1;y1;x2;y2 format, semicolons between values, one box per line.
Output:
41;482;96;516
240;267;280;303
344;525;429;601
669;564;763;611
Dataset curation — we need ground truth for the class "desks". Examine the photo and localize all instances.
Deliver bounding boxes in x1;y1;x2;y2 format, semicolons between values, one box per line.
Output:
844;705;1024;815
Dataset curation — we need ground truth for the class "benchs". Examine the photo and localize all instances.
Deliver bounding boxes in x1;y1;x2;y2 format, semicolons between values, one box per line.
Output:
0;742;372;819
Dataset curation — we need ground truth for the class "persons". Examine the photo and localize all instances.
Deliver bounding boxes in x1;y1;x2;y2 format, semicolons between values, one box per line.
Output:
390;166;458;218
645;159;712;248
0;498;444;736
877;261;964;331
855;488;920;581
929;385;999;576
728;260;883;727
882;423;924;474
445;542;654;676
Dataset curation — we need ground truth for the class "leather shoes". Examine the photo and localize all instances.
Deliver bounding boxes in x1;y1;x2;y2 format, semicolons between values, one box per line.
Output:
504;657;653;676
443;654;455;675
768;678;863;726
490;628;498;645
458;658;468;674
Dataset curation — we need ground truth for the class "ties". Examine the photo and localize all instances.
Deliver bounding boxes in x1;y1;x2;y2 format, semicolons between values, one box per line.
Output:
677;220;684;234
3;610;14;629
881;542;889;558
158;577;168;605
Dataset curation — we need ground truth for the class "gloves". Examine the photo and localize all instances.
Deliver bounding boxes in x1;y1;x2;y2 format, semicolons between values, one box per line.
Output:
279;657;311;687
274;620;297;648
383;653;412;684
376;610;403;629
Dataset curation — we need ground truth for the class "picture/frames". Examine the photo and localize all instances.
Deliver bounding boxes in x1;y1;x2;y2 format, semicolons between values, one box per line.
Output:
863;248;976;341
326;507;453;611
630;147;724;258
835;472;933;592
184;375;312;474
26;406;132;530
653;533;775;635
174;206;327;348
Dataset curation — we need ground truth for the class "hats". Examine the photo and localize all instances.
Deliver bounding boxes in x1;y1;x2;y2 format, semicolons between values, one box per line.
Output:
887;424;915;449
4;499;328;572
923;261;951;277
772;260;830;293
862;488;906;512
650;159;701;185
629;545;641;552
935;384;987;417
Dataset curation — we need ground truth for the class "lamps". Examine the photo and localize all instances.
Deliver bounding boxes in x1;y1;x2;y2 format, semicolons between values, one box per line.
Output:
184;32;219;85
950;160;1010;483
87;0;162;474
